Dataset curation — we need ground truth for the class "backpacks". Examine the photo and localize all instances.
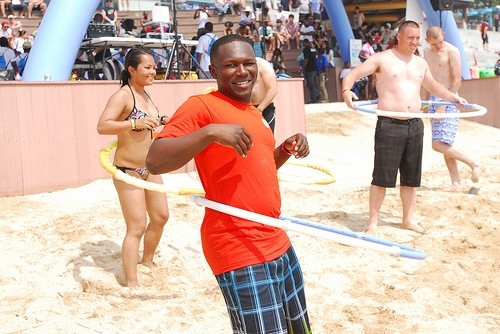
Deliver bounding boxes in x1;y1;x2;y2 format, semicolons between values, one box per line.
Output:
0;48;12;70
18;54;27;73
203;34;217;56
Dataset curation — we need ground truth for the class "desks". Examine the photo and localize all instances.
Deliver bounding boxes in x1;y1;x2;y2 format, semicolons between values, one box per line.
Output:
81;37;199;81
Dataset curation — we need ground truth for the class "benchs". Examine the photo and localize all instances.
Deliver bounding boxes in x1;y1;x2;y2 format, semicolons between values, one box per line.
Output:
94;7;319;102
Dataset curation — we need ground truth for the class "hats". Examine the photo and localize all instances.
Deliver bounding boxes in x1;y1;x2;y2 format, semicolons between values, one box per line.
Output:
21;42;32;49
243;5;253;12
239;20;246;26
2;22;10;27
8;15;14;19
278;4;283;7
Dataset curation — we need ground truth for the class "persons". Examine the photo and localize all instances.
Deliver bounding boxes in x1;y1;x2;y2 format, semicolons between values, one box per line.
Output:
353;6;365;28
249;56;278;134
340;20;398;100
188;3;219;80
96;44;170;287
140;11;152;38
342;21;468;235
77;13;111;80
422;25;479;189
120;19;137;38
214;0;237;15
0;0;48;81
224;21;234;35
144;34;311;334
479;18;489;50
238;0;338;102
102;0;118;24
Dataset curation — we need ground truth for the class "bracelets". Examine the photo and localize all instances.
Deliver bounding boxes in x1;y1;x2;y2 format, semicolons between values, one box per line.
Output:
131;119;135;128
257;108;263;112
342;89;349;94
92;51;96;56
161;116;166;125
281;143;292;155
